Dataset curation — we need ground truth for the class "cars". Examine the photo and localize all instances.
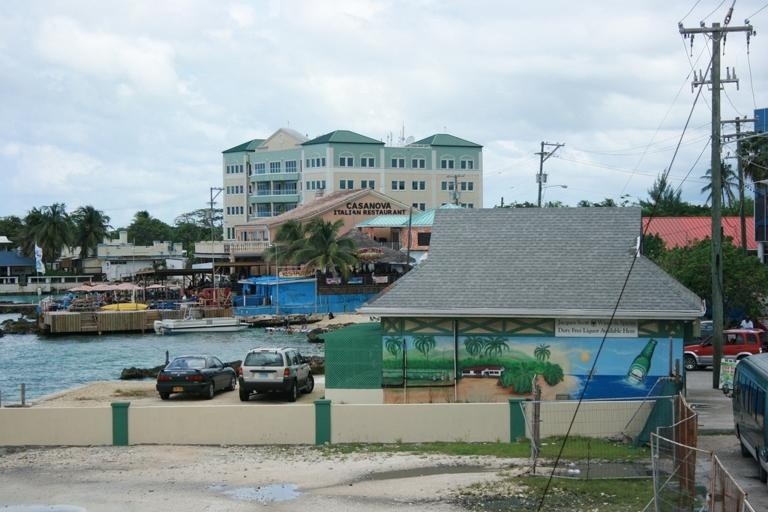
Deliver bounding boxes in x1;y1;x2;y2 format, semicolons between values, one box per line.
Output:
156;355;236;401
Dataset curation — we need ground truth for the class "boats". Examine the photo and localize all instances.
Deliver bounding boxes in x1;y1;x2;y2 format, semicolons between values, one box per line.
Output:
37;280;201;314
154;315;249;333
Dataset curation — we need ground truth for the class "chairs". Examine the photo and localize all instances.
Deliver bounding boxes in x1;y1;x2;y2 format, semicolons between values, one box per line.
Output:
191;308;204;319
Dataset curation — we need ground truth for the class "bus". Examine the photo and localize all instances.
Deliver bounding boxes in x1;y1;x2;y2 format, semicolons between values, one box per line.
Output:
729;352;768;477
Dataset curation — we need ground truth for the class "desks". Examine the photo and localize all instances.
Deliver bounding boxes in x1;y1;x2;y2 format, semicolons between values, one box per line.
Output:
173;302;203;321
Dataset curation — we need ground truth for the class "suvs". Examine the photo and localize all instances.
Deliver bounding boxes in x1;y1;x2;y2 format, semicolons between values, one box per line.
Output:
683;327;767;369
239;348;314;402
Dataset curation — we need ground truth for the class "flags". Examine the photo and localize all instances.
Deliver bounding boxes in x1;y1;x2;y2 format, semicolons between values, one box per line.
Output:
35;245;45;274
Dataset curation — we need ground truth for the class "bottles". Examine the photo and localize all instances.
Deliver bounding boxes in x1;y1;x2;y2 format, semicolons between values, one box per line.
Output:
625;338;658;387
